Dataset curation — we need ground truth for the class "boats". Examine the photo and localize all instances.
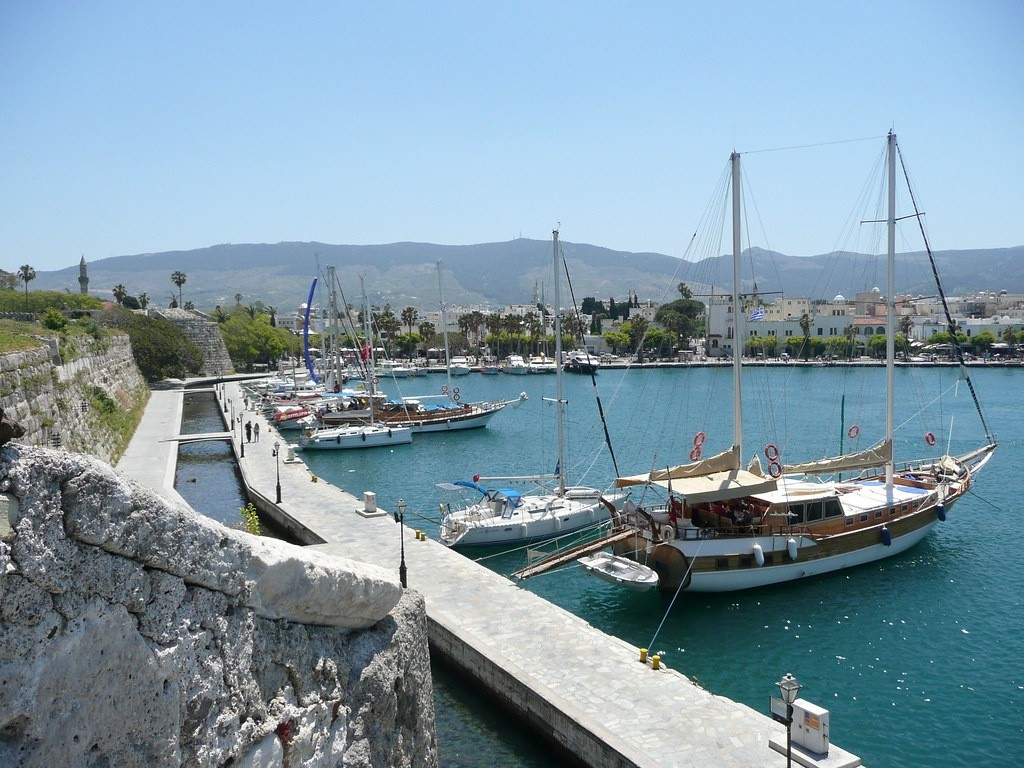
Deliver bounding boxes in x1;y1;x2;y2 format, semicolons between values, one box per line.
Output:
562;349;600;374
479;356;498;374
406;363;429;376
528;356;565;374
375;363;411;378
502;355;529;375
445;359;472;375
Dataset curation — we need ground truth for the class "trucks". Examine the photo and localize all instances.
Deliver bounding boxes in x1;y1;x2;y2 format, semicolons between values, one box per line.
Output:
276;357;298;367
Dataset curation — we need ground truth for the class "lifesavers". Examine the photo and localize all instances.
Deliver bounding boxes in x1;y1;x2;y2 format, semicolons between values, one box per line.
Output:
768;461;782;478
372;378;379;384
925;432;936;445
693;431;705;446
453;387;460;393
763;443;779;460
848;425;859;438
690;447;702;461
334;384;341;393
453;394;460;401
441;385;449;395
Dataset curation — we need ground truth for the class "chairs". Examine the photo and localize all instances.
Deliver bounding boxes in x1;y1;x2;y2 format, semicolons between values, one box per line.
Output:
698;508;739;534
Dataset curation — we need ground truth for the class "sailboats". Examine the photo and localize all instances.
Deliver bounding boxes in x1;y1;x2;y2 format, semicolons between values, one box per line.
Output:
510;129;998;591
296;258;529;432
251;254;387;429
439;230;633;546
298;296;413;449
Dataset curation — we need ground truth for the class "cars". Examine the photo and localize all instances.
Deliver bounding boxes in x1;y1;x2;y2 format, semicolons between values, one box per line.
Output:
781;353;790;360
756;353;768;360
601;354;618;359
917;353;937;360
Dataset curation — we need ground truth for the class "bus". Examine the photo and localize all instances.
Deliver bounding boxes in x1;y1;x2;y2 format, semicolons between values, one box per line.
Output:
415;348;452;365
315;359;333;369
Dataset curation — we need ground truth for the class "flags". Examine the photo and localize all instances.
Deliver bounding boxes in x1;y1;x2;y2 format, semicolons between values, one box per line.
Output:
748;307;763;322
554;460;560;479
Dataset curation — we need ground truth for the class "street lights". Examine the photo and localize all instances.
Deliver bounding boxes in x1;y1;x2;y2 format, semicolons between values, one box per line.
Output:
397;499;407;588
774;673;803;768
273;441;282;503
239;412;244;457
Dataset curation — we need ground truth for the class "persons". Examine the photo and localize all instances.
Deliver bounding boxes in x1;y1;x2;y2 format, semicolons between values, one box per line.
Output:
337;402;345;412
244;396;248;410
245;421;260;443
348;399;364;410
326;403;333;413
